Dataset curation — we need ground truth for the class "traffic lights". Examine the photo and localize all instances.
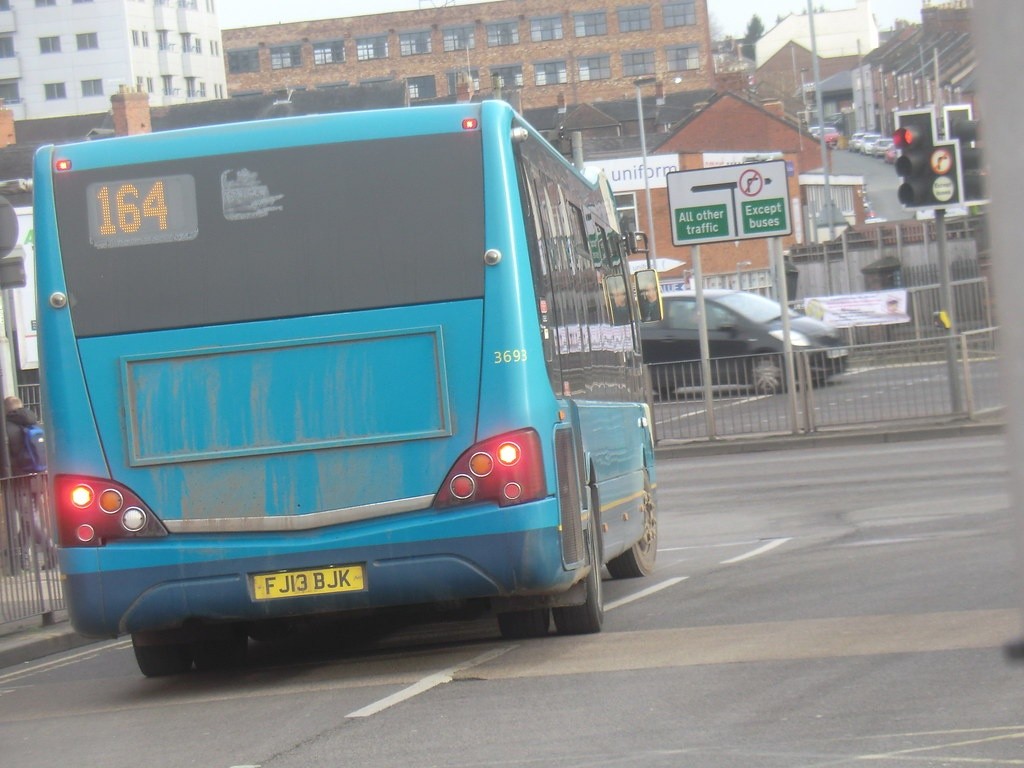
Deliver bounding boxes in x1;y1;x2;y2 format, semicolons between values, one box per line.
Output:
892;103;990;424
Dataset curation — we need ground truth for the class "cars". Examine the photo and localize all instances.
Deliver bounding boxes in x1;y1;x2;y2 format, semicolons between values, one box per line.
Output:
641;290;848;396
809;125;903;165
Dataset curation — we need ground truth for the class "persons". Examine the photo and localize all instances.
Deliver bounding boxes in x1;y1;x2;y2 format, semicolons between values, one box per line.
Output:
1;396;60;570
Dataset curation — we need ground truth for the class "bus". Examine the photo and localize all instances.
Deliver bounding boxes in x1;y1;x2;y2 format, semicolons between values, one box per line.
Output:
32;100;659;678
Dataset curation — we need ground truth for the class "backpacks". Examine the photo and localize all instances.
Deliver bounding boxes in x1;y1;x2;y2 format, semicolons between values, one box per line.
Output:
18;423;45;472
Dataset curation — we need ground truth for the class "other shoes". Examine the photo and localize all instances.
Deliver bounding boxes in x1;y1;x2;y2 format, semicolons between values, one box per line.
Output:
41;548;58;569
